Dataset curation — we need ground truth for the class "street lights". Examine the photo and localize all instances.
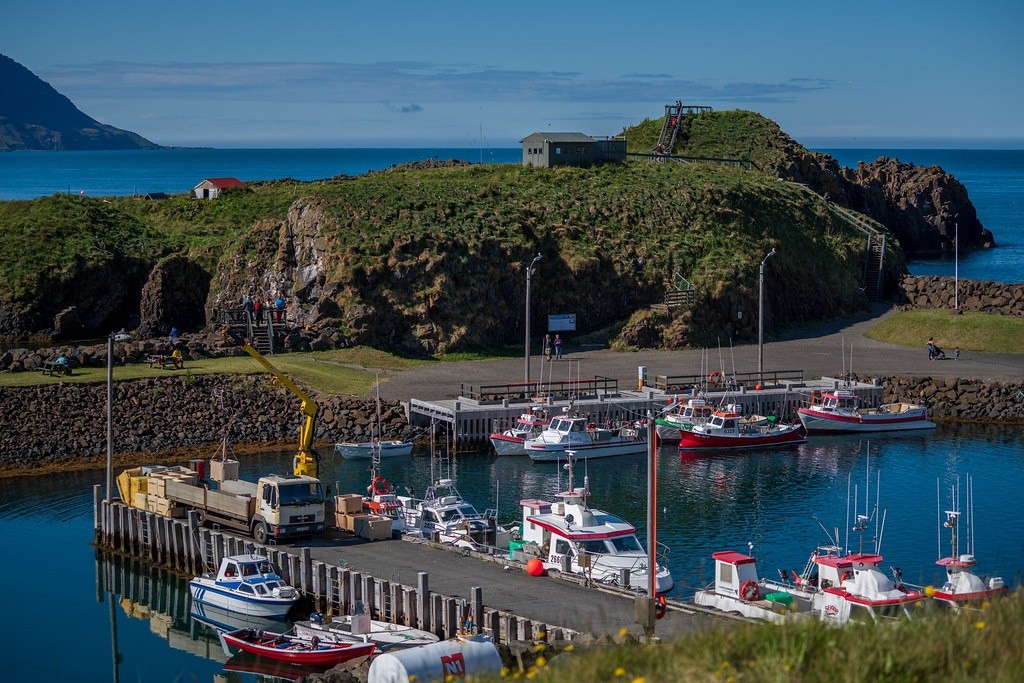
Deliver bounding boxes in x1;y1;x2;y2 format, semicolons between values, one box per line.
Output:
524;252;544;400
758;249;777;386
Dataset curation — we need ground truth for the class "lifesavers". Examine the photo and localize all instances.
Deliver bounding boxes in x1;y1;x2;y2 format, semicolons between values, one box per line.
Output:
372;477;390;495
741;581;760;601
840;571;854;582
709;370;721;383
654;594;667;620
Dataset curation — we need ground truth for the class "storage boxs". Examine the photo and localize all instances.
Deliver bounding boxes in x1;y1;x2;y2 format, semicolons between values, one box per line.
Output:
509;538;529;560
766;591;792;610
335;493;393;540
135;459;240;517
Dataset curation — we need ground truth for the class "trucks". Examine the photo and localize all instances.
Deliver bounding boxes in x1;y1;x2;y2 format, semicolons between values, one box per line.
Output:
165;462;326;543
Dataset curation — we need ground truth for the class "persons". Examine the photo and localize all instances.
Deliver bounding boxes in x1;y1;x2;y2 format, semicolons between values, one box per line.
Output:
392;484;396;492
927;337;936;361
275;294;287;323
405;487;415;497
254;299;264;326
671;118;677;128
169;327;179;339
892;568;902;588
156;354;166;369
55;354;68;375
227;312;247;324
657;144;662;153
554;335;562;360
955;347;960;360
545;335;552;362
172;348;184;368
245;297;255;323
675;101;682;107
266;297;274;324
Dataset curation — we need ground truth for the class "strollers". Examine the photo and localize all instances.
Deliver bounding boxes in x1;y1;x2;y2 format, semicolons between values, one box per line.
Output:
932;345;946;360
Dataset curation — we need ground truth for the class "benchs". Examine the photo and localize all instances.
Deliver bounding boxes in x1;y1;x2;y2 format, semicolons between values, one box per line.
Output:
37;366;77;372
318;646;333;650
277;642;293;649
145;358;181;364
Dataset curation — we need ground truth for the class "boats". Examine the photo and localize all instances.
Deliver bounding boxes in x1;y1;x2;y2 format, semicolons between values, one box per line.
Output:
359;428;675;593
489;338;648;460
796;337;937;434
692;440;1013;629
106;328;135;503
221;652;331;683
190;552;441;665
335;373;414;459
654;337;808;454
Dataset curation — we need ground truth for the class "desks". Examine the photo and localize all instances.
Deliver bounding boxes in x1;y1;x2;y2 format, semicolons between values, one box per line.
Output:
148;354;179;369
42;361;69;376
